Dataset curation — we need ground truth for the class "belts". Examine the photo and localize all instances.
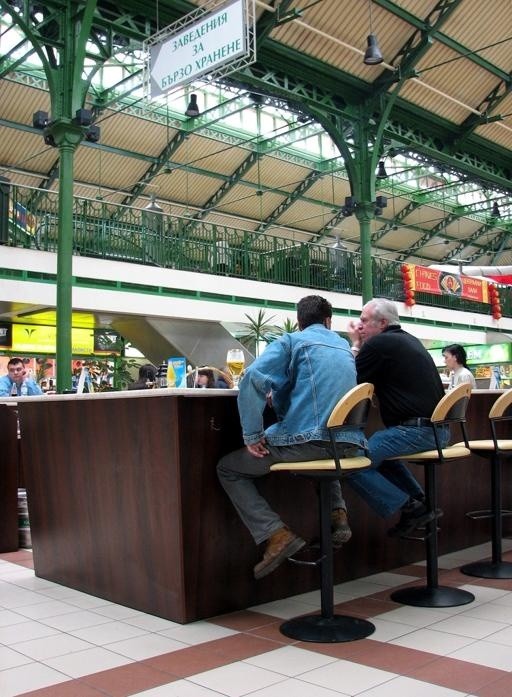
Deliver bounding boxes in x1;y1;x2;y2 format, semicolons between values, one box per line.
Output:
401;417;450;429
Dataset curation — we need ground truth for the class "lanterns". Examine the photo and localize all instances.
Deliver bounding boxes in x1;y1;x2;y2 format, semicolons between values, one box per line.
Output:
488;284;501;320
401;265;416;307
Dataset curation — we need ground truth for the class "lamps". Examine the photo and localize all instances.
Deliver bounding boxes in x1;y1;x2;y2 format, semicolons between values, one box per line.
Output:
184;93;200;117
491;200;500;218
375;160;388;181
361;1;385;65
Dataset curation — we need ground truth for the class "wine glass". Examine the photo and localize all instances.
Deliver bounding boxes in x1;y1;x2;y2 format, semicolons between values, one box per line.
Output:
227;349;245;390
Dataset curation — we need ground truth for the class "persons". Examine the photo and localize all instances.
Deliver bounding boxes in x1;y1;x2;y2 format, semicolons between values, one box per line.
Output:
214;293;370;578
439;343;477;390
197;365;214;389
127;364;157;391
207;236;393;296
217;367;230;388
0;357;44;397
346;297;449;544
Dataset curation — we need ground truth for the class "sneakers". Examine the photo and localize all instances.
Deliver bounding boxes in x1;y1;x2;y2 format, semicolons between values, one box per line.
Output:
386;496;446;540
254;525;306;581
308;508;353;549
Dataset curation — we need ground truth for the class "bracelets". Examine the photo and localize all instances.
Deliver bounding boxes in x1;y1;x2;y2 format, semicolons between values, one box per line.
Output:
349;346;360;353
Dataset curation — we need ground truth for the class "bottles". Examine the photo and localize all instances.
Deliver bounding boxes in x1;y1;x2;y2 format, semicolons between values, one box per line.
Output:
160;360;167;387
168;361;176;387
21;381;27;396
11;383;17;396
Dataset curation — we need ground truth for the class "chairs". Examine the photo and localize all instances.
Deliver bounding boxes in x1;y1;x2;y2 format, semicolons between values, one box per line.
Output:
449;387;512;579
386;382;476;607
269;382;376;642
